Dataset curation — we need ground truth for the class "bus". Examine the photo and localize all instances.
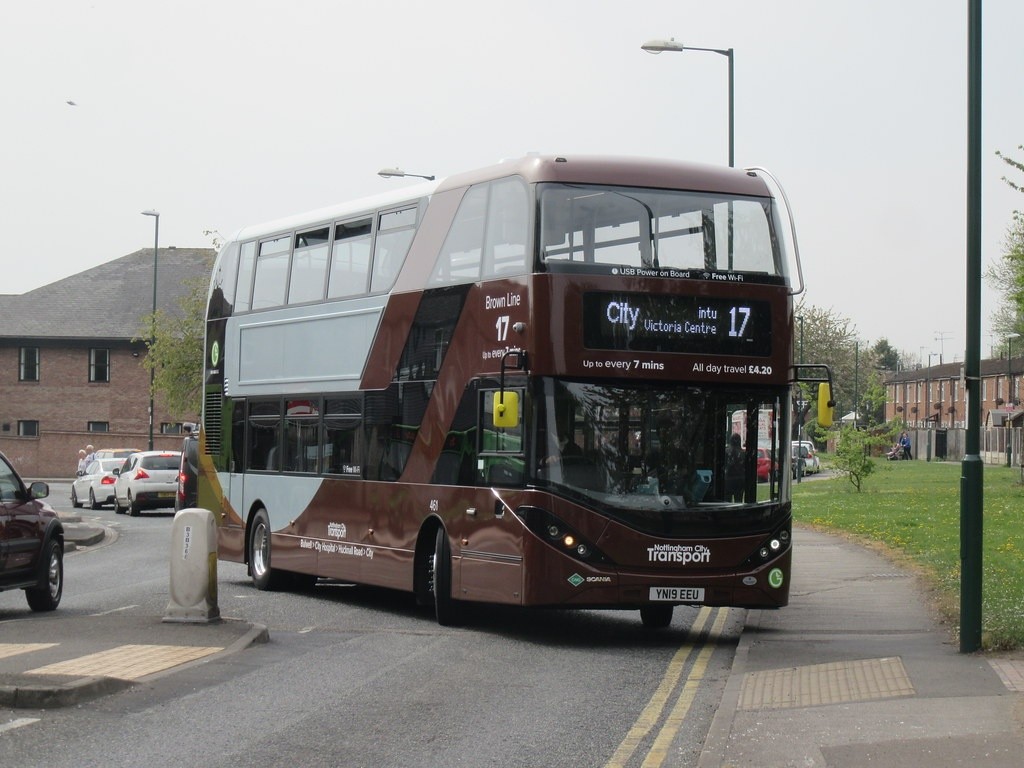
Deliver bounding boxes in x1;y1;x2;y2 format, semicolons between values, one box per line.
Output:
196;152;837;631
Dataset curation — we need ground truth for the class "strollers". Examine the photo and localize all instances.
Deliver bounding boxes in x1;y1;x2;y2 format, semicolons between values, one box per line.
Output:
885;443;905;461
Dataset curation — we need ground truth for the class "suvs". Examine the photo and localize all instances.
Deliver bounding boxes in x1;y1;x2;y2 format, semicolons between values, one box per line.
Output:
0;450;65;610
112;450;182;517
95;448;143;459
174;422;201;513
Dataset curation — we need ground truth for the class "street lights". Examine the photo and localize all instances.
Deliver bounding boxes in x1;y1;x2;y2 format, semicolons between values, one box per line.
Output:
1005;331;1021;467
141;208;161;451
639;35;737;441
793;314;805;483
849;339;858;430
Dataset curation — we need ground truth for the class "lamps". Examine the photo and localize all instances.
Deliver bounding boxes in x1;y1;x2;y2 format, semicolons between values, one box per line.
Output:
132;351;139;357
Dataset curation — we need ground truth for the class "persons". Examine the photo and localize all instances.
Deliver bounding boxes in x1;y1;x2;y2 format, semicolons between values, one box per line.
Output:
726;433;747;503
900;432;913;460
78;449;86;474
85;445;95;466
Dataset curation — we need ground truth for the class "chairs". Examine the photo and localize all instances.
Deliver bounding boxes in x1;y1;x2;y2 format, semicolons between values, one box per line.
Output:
267;445;295;470
251;441;270;469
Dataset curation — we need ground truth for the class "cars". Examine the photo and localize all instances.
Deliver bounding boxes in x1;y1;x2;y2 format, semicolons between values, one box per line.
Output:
771;440;821;478
742;447;779;482
70;458;127;510
